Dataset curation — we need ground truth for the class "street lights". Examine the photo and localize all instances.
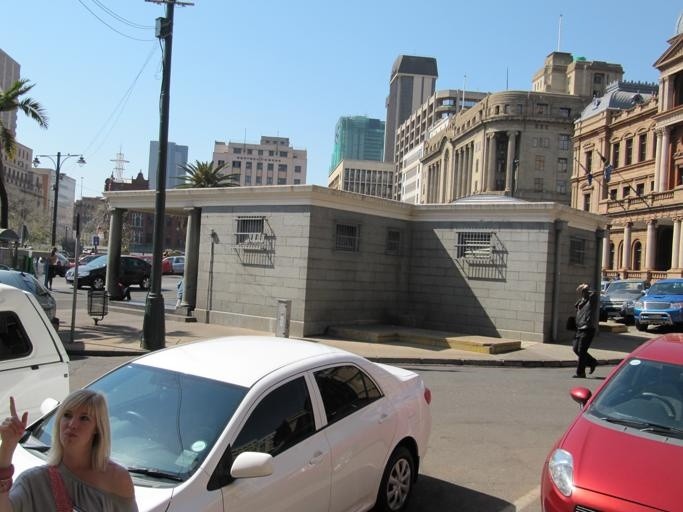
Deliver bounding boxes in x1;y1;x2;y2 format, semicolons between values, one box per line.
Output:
32;153;85;287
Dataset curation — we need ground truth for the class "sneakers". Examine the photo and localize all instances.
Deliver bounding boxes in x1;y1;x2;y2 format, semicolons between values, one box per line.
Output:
573;372;586;378
589;358;599;374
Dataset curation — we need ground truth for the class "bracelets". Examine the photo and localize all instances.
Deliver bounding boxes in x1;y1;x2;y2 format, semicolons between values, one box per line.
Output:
0;464;14;479
0;477;14;493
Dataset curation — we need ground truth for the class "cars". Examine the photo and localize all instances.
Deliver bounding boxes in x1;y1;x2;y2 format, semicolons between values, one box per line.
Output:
64;250;185;295
0;336;432;512
540;332;682;510
598;281;627;294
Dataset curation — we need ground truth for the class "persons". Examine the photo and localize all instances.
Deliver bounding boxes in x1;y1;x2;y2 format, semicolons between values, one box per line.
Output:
164;251;169;257
45;247;57;290
0;389;138;512
119;282;131;300
176;272;184;306
571;284;599;379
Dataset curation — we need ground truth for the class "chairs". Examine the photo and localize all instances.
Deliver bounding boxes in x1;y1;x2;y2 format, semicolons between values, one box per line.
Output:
631;366;683;403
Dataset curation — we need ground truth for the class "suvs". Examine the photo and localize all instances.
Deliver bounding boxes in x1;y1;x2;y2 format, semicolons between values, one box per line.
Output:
0;283;74;438
634;279;683;332
0;266;62;335
599;279;651;324
